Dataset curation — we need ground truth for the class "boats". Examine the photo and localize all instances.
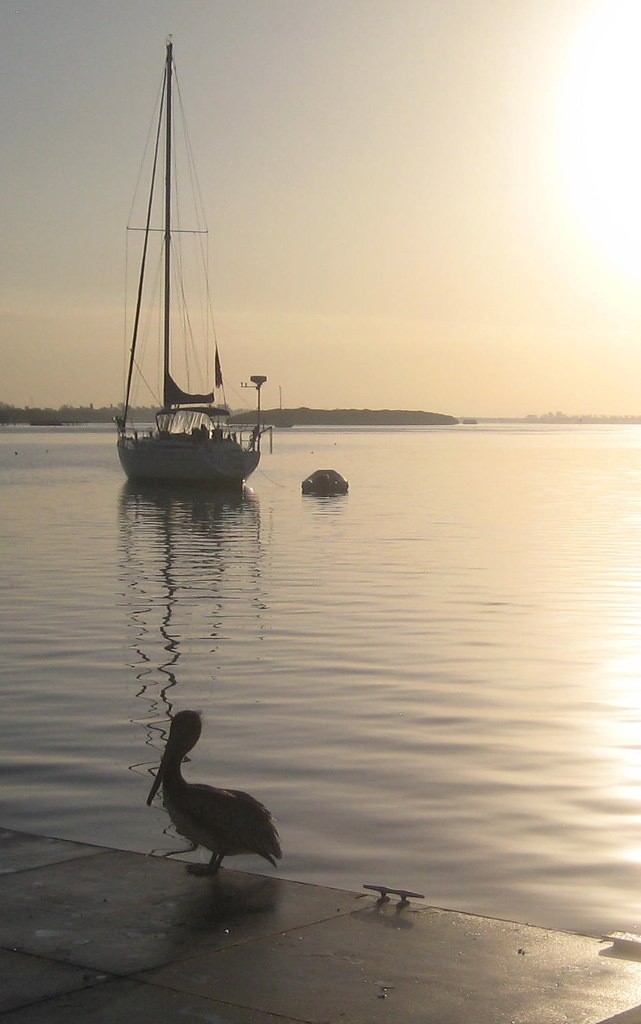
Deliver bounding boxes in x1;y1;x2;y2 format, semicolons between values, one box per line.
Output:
301;470;349;496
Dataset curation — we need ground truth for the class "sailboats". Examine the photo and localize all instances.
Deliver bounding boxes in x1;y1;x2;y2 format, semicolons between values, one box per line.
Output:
275;386;293;428
113;34;272;490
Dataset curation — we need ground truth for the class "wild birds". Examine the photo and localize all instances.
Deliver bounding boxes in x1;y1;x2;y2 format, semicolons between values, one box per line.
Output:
146;709;285;879
